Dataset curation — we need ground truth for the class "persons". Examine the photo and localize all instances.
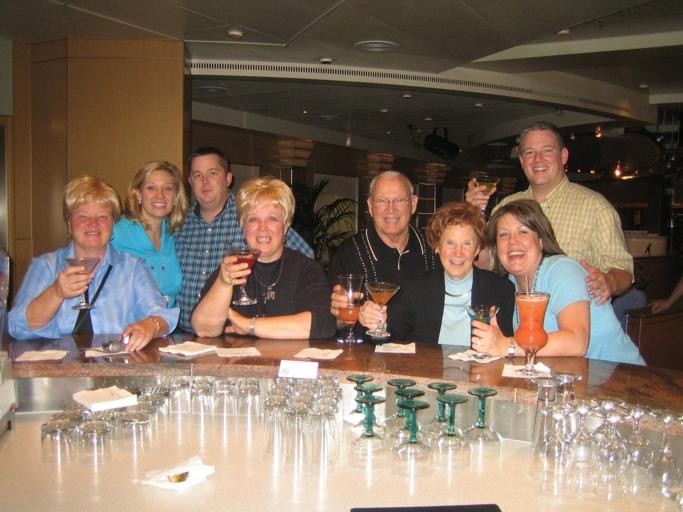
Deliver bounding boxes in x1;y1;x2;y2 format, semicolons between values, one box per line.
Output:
470;199;647;366
191;172;336;339
611;259;647;332
649;275;683;314
465;120;635;306
358;201;516;347
328;170;441;343
171;147;316;336
7;174;181;352
109;160;190;309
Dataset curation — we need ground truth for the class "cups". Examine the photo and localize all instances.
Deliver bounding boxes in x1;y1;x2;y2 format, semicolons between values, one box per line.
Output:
40;376;344;467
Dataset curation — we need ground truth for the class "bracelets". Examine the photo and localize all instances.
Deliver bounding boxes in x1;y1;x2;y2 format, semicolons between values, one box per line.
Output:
249;317;256;335
149;317;160;338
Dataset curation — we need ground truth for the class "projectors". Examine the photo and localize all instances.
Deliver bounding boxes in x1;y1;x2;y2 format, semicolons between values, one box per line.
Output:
424;134;460;161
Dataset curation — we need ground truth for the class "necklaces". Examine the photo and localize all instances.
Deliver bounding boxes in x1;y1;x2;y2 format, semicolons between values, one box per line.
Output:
252;255;284;302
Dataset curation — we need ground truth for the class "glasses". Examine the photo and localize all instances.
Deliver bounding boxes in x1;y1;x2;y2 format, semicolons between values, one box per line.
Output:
372;192;411;208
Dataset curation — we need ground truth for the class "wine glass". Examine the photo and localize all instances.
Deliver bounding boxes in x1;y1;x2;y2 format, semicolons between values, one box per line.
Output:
229;248;261;307
63;257;101;310
466;290;551;378
344;372;682;501
335;273;401;345
